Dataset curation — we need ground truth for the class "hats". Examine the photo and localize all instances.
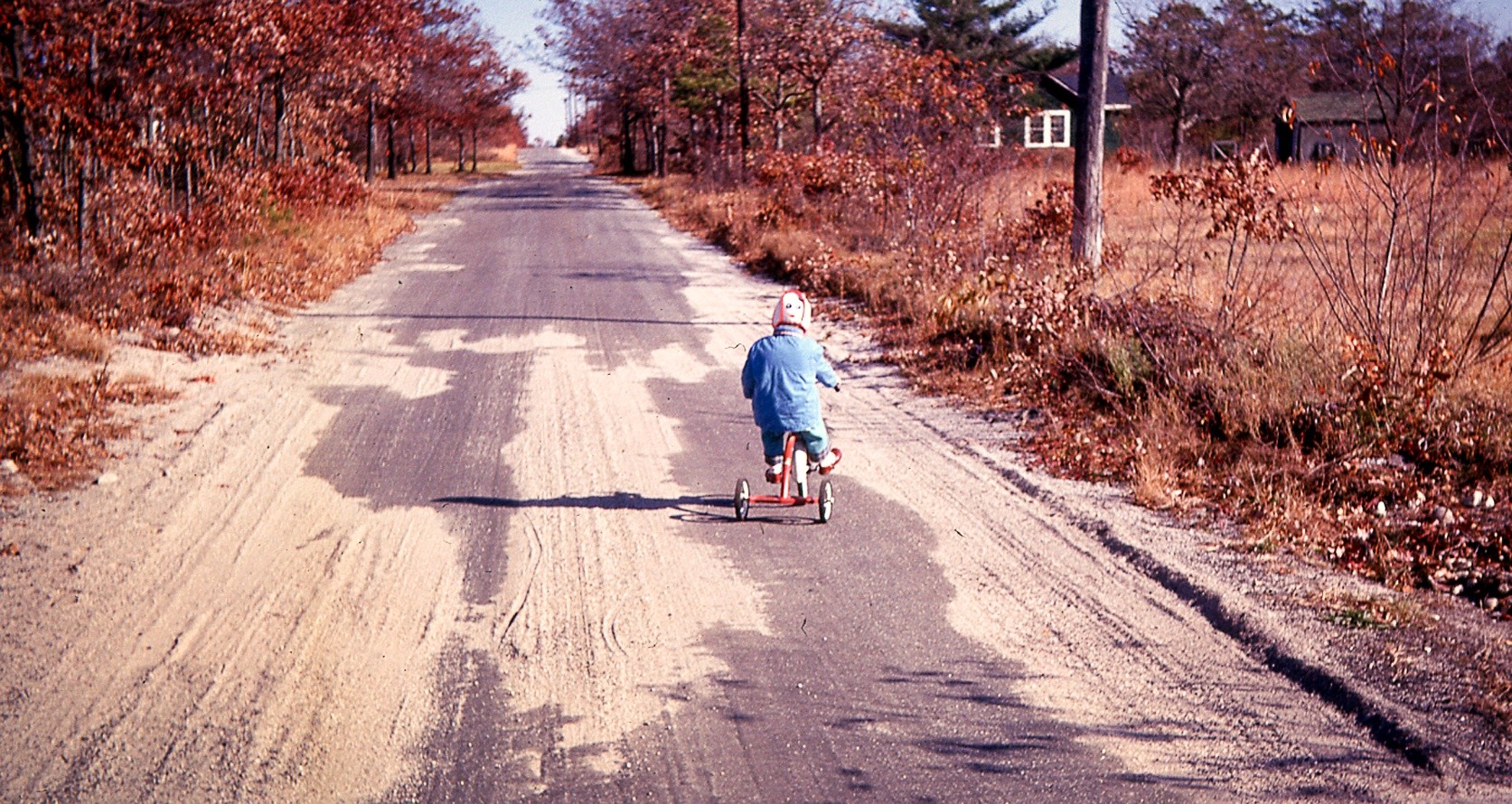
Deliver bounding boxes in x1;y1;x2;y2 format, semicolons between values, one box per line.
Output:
772;289;811;331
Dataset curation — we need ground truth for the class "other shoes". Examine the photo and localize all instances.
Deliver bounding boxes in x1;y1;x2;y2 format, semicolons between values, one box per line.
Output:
819;449;842;474
765;469;781;483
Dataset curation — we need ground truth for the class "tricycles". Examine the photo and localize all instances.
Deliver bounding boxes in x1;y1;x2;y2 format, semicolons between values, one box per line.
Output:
734;379;844;522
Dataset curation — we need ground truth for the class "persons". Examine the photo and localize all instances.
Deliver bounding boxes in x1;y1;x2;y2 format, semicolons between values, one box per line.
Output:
737;285;839;484
